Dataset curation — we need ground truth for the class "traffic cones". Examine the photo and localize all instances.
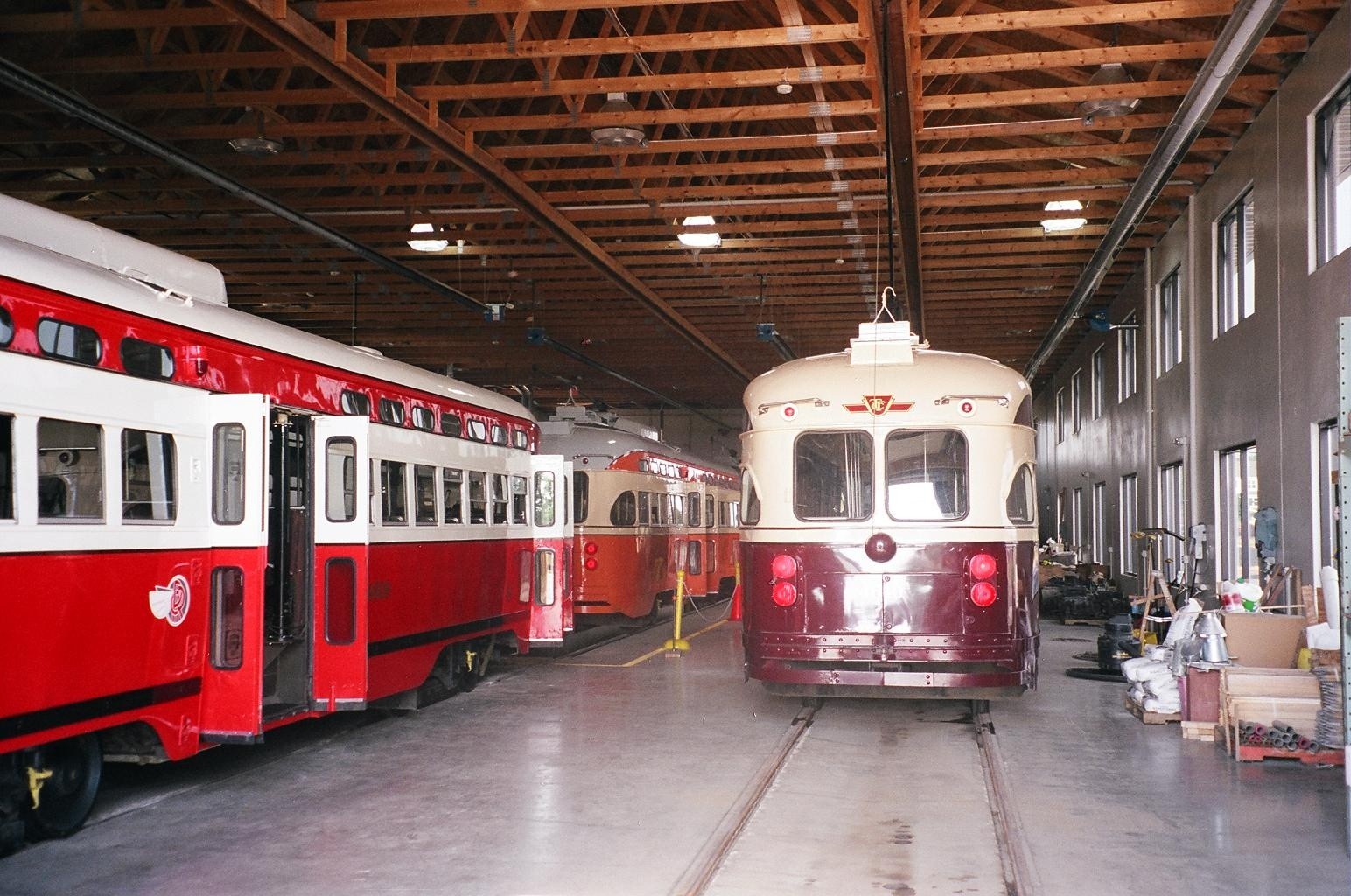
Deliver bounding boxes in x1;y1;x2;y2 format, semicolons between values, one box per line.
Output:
727;584;742;621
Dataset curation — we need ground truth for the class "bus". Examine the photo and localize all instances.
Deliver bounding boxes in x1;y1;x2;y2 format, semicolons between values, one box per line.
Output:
0;191;575;854
735;287;1042;699
531;386;741;651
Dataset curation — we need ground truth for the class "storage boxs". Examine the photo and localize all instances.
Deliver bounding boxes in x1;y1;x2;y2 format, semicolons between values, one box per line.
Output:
1221;611;1306;668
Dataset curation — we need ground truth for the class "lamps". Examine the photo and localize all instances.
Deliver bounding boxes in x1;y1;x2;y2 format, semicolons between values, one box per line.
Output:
407;222;450;252
1082;470;1089;477
590;91;647;147
1173;435;1186;446
229;108;285;155
677;216;722;248
1040;200;1086;231
1077;22;1143;118
